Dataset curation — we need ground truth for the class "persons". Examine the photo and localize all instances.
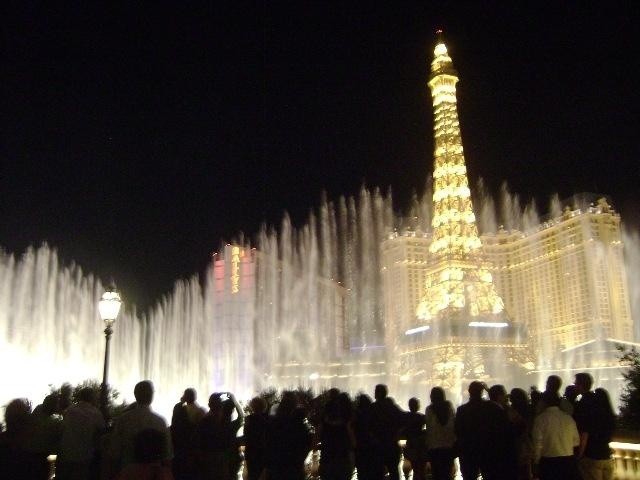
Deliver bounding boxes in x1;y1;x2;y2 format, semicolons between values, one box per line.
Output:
171;384;404;479
405;371;619;480
0;380;173;480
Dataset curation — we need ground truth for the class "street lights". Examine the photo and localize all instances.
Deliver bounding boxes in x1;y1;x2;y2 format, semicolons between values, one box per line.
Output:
97;284;123;427
